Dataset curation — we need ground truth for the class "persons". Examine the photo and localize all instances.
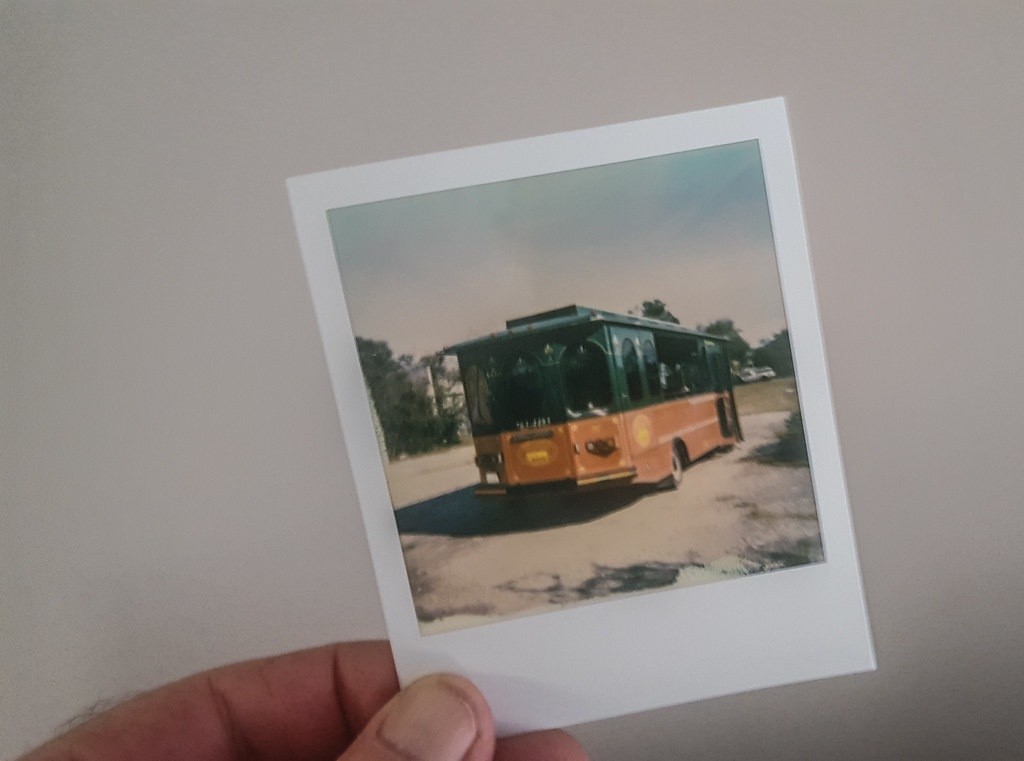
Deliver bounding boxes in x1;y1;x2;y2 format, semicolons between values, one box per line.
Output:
21;640;586;761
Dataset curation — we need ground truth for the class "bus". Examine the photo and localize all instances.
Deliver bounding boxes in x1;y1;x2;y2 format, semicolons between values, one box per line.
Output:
443;301;749;503
